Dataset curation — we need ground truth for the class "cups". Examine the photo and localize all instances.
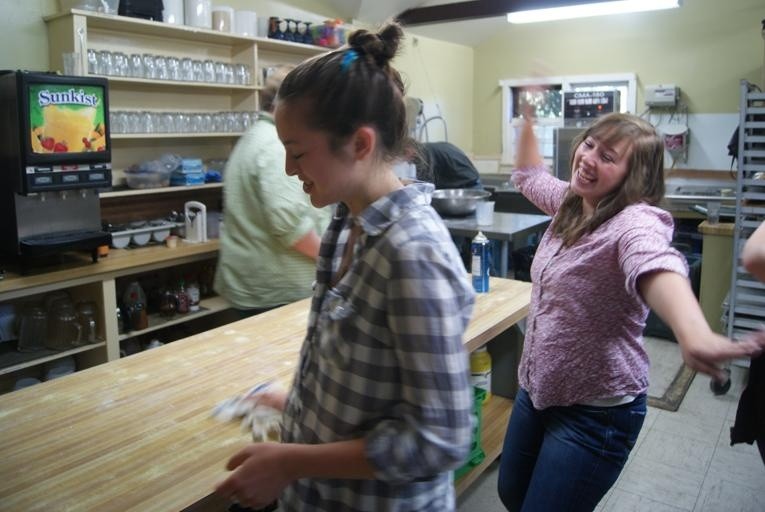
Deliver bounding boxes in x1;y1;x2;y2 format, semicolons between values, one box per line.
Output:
707;201;721;225
109;110;260;134
16;292;100;354
476;201;496;226
87;48;250;86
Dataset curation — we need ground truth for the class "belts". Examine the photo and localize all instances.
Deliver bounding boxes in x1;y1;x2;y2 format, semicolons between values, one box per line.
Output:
457;179;480;188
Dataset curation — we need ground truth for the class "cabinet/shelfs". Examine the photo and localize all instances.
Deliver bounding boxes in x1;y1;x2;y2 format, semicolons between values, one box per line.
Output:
726;76;765;368
42;8;339;201
220;311;533;512
0;246;282;395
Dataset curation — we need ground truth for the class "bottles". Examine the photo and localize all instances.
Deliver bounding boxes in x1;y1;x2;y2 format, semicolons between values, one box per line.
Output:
157;279;201;321
472;231;490;294
470;346;492;405
117;281;148;335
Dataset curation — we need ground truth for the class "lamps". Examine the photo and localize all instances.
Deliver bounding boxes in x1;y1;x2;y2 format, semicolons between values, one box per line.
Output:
506;0;681;24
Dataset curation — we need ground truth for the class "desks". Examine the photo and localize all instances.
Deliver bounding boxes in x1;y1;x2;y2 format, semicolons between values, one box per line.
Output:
443;211;553;279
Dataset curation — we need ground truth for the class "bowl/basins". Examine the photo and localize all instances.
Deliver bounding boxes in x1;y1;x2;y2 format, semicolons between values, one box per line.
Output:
431;188;493;219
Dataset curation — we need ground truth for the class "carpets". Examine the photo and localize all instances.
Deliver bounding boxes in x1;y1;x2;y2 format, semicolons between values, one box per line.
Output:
641;333;698;411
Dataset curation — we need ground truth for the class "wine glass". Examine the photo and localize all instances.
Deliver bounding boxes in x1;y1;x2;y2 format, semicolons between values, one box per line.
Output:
274;18;314;45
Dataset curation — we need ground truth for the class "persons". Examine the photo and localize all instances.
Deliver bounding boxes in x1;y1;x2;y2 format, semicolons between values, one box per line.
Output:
212;18;475;511
724;217;765;465
494;51;765;512
214;59;335;314
